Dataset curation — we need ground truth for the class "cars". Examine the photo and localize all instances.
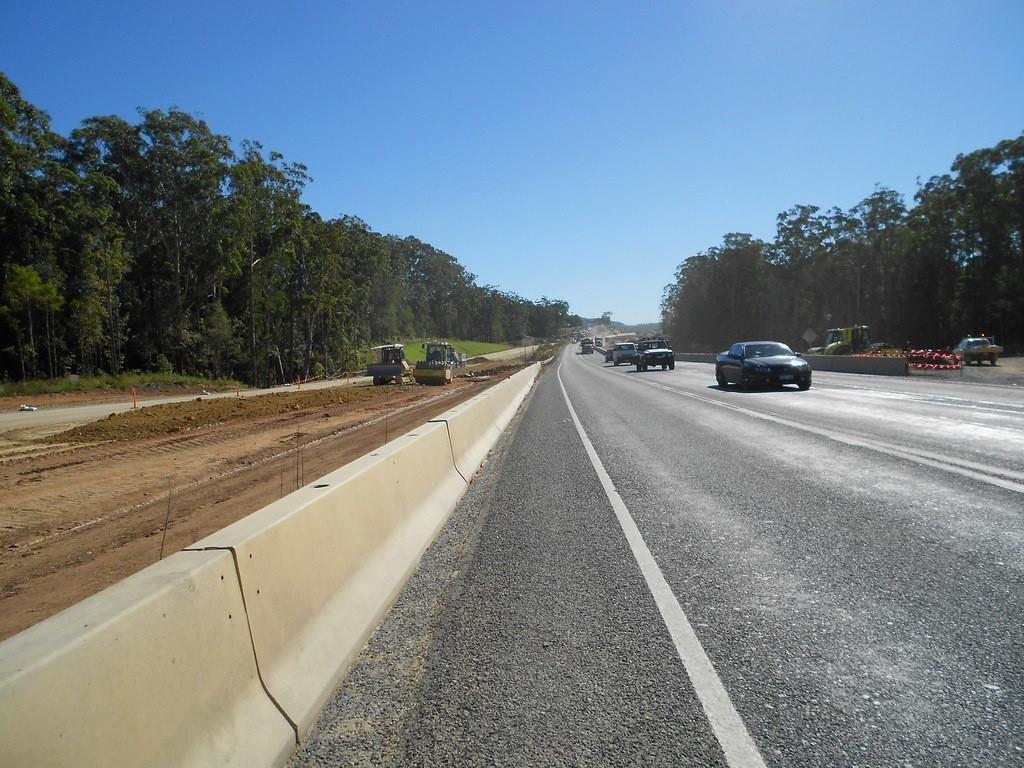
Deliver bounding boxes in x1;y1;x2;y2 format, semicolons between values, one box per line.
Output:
605;348;616;362
715;341;812;389
954;338;1003;365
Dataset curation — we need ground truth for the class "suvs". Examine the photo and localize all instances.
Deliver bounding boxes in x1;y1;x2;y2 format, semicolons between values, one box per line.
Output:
636;338;674;372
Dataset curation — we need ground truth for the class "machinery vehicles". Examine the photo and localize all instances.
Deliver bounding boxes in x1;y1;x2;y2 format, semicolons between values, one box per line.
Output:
807;324;894;354
366;343;411;387
413;341;454;385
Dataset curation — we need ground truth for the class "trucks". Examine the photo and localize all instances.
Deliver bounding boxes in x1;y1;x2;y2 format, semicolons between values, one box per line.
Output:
595;337;603;346
580;337;594;354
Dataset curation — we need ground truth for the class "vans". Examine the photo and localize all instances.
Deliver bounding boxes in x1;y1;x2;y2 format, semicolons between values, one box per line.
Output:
613;343;637;366
